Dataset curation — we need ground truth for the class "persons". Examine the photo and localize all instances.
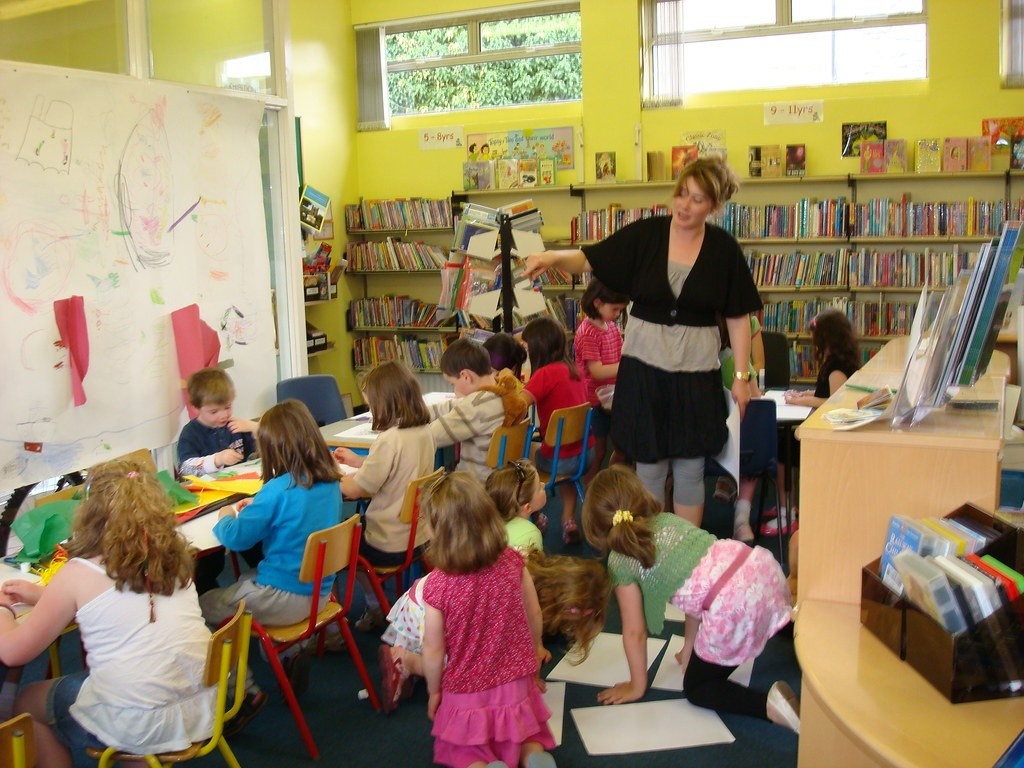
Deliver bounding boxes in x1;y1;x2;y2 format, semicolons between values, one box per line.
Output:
420;472;561;768
758;309;861;536
580;466;800;736
301;360;438;656
201;398;343;716
176;367;261;596
519;154;766;531
0;459;224;768
573;277;630;485
718;303;765;542
431;315;598;545
381;456;609;711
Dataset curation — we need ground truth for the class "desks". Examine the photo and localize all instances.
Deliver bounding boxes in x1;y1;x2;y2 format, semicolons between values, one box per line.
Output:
321;388;501;465
0;450;367;677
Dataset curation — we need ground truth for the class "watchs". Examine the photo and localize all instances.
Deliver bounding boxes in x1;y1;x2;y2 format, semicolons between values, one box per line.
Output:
733;371;754;383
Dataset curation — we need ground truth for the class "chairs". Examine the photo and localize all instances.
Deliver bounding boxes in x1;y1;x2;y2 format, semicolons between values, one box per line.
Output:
0;712;42;768
482;415;545;492
735;398;785;578
761;331;790;390
529;400;600;509
272;375;349;425
253;513;380;755
83;599;253;767
369;467;456;615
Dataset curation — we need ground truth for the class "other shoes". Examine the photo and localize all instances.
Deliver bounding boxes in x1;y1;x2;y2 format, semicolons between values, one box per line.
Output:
301;626;348;651
379;643;410;716
562;521;582;544
354;605;388;634
535;514;549;533
760;517;798;536
766;681;800;734
282;652;310;702
733;524;755;545
713;477;730;504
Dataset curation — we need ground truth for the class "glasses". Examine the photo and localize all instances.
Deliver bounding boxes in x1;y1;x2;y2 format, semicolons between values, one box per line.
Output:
508;461;526;502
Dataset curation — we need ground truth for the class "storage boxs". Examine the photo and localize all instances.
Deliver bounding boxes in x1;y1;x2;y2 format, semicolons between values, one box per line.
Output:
748;144;781;178
305;322;327;354
861;135;992;176
857;499;1024;703
303;276;338;302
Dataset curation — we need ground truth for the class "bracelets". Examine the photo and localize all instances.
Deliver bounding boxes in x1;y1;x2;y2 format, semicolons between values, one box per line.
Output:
0;603;16;620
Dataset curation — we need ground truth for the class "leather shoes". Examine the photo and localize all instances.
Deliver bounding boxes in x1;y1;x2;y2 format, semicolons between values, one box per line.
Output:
763;503;779;517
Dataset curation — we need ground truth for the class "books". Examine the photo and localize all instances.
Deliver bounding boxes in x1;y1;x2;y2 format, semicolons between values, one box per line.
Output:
529;135;1024;387
343;196;460;375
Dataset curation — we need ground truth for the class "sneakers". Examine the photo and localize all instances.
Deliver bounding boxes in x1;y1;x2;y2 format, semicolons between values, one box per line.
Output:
224;680;269;738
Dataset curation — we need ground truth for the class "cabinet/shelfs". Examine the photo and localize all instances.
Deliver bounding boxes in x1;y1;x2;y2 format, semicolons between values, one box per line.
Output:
344;175;1024;384
